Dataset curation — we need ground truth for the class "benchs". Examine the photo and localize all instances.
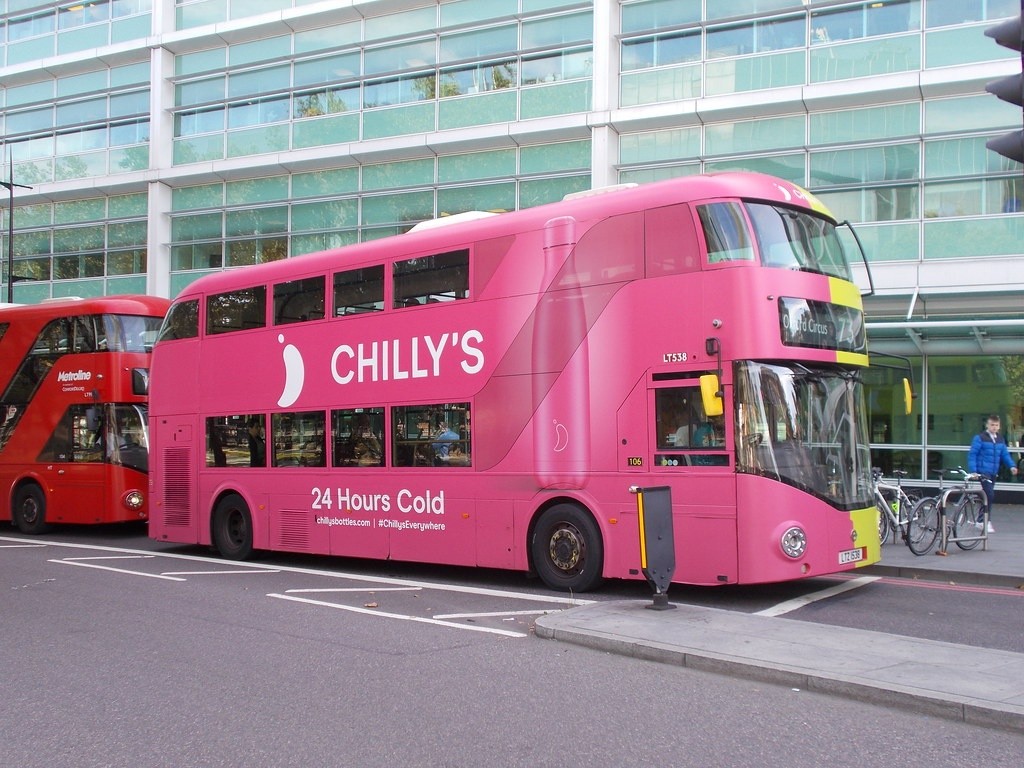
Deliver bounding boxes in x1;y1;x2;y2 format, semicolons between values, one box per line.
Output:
211;293;468;336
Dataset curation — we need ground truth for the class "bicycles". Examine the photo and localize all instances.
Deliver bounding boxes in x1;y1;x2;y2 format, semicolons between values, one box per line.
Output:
873;469;927;543
908;466;993;555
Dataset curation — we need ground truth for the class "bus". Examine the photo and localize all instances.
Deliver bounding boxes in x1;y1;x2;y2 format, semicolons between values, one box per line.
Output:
0;297;174;534
865;357;1014;476
148;174;917;592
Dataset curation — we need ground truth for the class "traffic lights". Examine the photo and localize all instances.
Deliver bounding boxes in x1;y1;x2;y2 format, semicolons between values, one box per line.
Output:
983;0;1024;163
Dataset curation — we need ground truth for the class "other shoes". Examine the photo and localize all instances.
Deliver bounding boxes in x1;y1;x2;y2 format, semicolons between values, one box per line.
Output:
977;521;994;533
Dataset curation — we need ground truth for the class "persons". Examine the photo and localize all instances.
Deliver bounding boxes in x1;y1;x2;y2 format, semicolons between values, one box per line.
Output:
966;413;1018;533
238;419;266;468
673;399;726;467
431;418;461;464
